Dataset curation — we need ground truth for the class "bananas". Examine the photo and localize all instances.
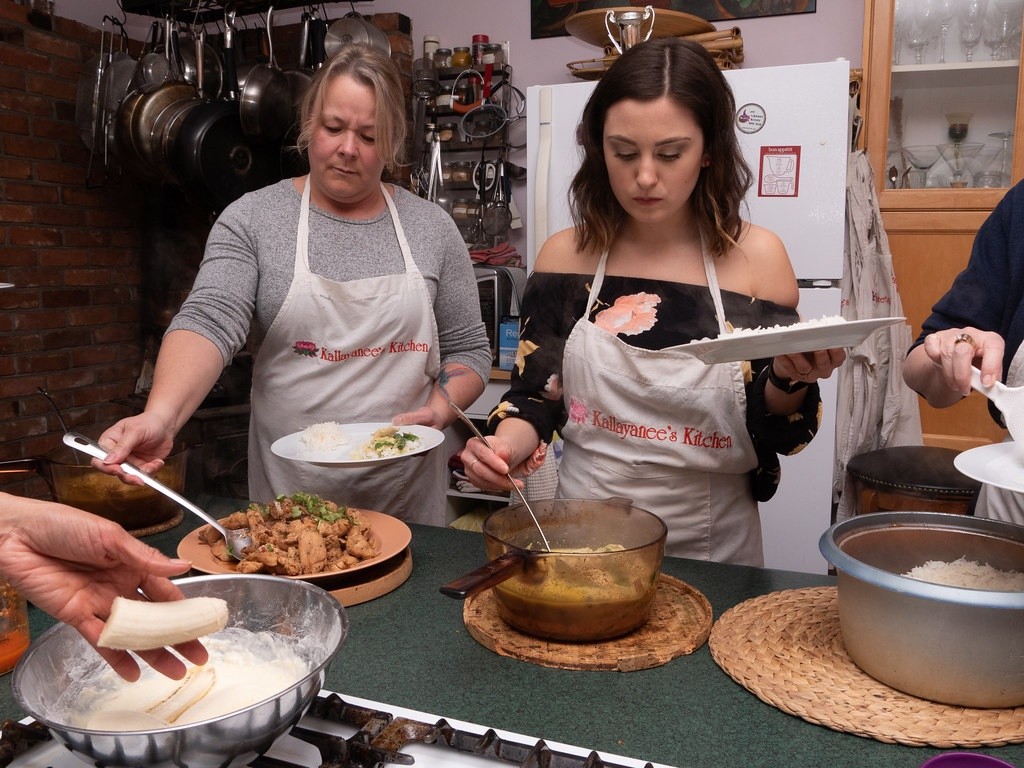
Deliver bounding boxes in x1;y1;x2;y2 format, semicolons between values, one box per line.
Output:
87;710;169;731
96;595;228;650
147;664;216;723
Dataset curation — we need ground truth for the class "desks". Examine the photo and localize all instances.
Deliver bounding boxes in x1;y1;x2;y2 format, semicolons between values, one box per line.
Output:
405;378;512;529
108;392;254;500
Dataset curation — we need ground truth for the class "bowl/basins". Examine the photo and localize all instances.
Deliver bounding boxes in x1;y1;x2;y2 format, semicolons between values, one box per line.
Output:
12;574;349;768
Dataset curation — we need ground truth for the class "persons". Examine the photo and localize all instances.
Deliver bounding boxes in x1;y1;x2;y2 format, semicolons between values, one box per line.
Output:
0;489;209;684
900;178;1024;528
459;36;848;571
89;39;495;531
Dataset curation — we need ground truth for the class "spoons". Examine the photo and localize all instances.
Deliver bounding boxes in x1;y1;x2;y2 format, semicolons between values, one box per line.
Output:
900;166;912;189
888;165;898;189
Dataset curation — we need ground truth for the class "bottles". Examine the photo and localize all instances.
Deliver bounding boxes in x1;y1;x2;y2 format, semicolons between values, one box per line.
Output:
441;159;498;182
442;76;480;106
436;34;504;69
450;198;482;218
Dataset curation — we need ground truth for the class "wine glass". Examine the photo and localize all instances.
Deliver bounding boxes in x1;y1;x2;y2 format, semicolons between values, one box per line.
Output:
936;143;985;181
893;0;1024;65
902;145;948;188
988;132;1012;188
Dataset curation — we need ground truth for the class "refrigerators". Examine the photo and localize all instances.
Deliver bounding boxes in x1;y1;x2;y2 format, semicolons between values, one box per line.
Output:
526;57;852;576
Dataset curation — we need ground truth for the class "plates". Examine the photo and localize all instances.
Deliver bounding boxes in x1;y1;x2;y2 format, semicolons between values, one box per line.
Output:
177;509;411;580
271;422;446;467
953;441;1024;493
659;317;909;364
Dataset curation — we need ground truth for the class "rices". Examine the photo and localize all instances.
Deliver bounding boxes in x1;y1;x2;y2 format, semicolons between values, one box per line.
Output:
299;420;350;451
690;314;846;343
898;556;1024;592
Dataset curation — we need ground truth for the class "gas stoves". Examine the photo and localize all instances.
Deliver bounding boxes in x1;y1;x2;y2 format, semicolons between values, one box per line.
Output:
0;676;679;768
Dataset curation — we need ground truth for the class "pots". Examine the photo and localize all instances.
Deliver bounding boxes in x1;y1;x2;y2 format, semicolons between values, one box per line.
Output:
439;496;667;643
819;511;1024;708
114;6;391;225
0;438;191;531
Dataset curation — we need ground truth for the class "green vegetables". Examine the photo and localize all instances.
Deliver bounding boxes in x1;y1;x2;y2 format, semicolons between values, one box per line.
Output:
247;491;356;527
373;430;418;451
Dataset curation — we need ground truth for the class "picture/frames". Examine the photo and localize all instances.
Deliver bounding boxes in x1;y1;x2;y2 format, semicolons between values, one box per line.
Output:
531;0;817;40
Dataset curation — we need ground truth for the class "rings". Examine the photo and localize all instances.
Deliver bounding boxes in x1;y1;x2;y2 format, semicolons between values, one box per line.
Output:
953;333;979;352
470;458;480;469
796;367;814;381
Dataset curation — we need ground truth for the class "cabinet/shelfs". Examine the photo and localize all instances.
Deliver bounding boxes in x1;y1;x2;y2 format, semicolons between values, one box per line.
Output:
419;65;513;381
857;0;1024;448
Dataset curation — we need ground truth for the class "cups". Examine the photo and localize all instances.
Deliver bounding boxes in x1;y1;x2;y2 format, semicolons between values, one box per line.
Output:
972;172;1002;189
904;171;926;188
946;112;974;139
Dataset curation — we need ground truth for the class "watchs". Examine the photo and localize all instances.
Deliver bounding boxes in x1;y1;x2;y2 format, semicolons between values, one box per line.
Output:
767;357;810;396
